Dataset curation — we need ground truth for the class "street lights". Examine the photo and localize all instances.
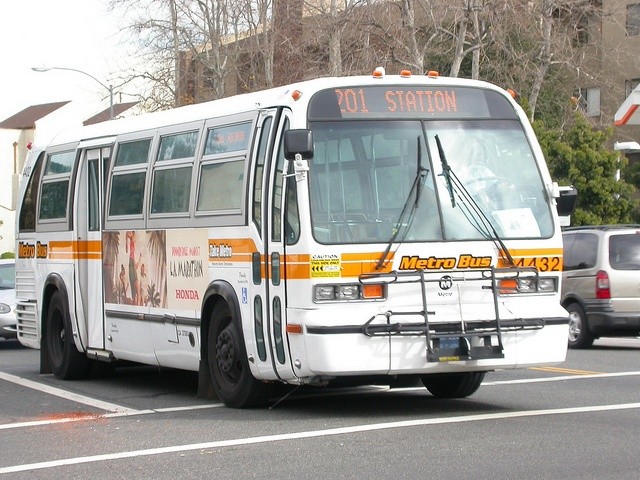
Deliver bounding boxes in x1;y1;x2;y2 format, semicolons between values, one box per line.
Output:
32;66;114;119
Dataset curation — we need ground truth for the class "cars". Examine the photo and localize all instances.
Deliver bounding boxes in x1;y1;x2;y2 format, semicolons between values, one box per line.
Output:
0;259;15;343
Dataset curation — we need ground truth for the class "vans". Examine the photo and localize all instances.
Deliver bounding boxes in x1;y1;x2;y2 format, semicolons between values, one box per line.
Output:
563;224;640;349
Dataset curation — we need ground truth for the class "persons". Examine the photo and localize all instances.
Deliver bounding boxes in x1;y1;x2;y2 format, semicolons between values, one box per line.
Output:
137;264;147;306
419;143;516;215
119;264;130;298
126;231;140;304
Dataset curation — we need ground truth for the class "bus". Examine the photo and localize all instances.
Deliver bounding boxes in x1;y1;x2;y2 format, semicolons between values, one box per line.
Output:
14;67;572;410
